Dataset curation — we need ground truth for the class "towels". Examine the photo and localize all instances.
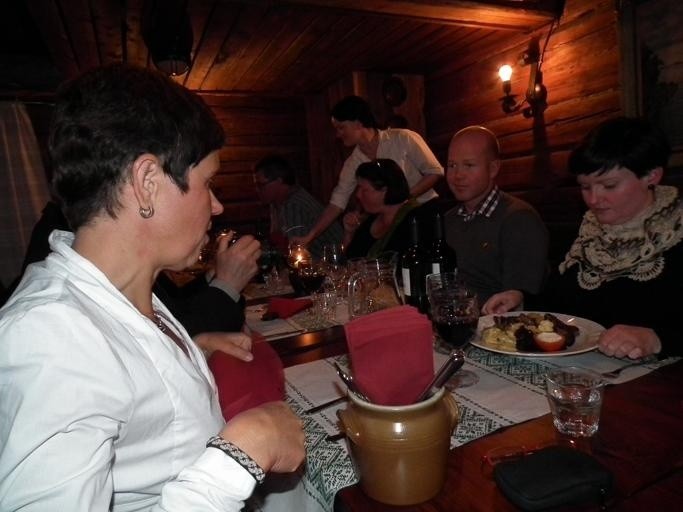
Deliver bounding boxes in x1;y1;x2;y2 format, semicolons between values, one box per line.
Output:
204;335;289;425
262;292;314;320
341;302;436;406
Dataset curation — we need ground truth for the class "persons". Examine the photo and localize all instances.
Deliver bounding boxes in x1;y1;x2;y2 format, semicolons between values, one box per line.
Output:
288;96;444;256
25;195;261;337
251;154;346;253
2;66;310;512
339;155;421;264
483;112;683;360
430;125;549;315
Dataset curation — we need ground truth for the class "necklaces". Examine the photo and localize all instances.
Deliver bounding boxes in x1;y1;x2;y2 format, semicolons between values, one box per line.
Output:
146;314;167;336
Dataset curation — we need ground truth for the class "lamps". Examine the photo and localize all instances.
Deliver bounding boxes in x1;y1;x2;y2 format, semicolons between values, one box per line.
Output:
498;63;523;112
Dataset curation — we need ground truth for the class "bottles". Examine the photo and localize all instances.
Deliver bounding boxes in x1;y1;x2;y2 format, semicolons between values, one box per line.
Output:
400;214;428;311
429;213;458;292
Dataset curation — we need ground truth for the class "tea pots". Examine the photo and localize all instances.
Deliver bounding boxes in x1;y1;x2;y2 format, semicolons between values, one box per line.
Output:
348;249;405;320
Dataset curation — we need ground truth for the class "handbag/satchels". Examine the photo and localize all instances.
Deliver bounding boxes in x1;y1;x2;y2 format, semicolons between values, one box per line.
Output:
494;445;611;512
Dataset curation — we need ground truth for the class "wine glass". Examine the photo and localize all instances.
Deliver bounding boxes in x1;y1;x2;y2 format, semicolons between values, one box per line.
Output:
425;274;481;390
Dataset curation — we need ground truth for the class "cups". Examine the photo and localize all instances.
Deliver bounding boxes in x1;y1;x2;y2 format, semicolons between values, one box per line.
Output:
206;229;234;255
257;242;358;325
544;364;603;438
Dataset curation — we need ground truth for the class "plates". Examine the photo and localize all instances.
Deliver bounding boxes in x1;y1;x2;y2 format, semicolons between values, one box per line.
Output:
466;310;608;360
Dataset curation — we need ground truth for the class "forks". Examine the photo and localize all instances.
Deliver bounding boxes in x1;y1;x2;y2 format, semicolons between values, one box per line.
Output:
593;357;661;378
